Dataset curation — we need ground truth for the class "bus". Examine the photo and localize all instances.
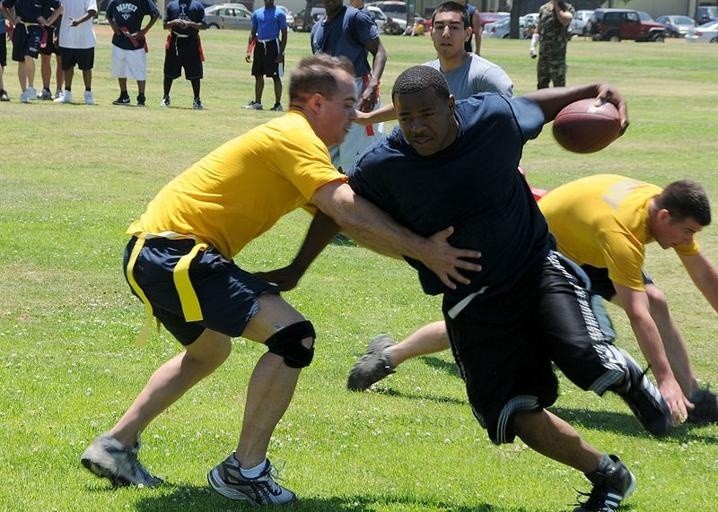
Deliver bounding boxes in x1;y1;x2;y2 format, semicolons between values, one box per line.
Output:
372;2;408;19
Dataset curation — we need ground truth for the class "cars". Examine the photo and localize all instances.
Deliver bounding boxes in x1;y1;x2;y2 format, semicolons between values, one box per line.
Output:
201;5;252;30
479;12;501;28
686;20;718;41
568;10;595;35
292;8;327;31
277;5;293;24
655;15;698;39
365;6;407;32
483;16;536;39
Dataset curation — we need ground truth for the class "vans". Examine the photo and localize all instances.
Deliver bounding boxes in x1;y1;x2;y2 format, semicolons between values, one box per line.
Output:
690;5;718;25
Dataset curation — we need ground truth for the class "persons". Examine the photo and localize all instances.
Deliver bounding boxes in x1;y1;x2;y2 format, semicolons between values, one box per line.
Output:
457;0;482;57
0;0;64;103
160;0;209;111
347;173;718;427
239;0;288;112
247;65;676;512
350;0;514;125
79;53;483;508
53;0;99;106
0;15;14;102
105;0;160;107
37;0;65;102
310;0;388;174
349;0;378;30
529;0;575;88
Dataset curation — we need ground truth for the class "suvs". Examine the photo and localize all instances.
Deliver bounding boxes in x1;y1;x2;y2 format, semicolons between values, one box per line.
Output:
585;9;665;42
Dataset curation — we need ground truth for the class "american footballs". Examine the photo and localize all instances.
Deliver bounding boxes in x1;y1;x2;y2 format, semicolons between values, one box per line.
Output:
552;96;625;155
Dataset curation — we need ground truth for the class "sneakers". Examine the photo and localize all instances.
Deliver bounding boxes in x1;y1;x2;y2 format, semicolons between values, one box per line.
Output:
20;92;27;102
59;90;72;102
206;453;296;506
137;96;146;105
0;89;9;101
38;89;51;100
193;98;203;109
241;101;263;110
160;98;170;107
565;453;636;512
84;91;94;104
29;87;37;100
347;333;398;390
79;434;162;488
112;95;130;105
54;91;62;98
270;103;282;111
618;349;670;440
684;389;718;424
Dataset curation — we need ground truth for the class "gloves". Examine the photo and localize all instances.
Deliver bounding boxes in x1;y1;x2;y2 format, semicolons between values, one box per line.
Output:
529;47;537;59
